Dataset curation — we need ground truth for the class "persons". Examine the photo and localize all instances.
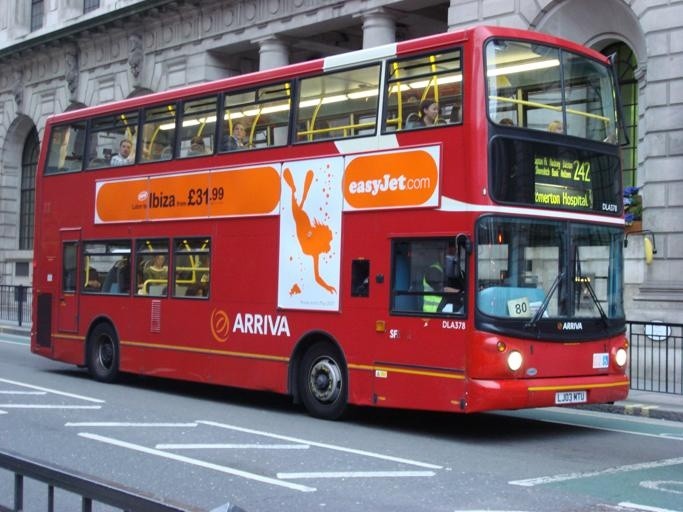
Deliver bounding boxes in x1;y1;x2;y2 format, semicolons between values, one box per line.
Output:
449;103;462;124
116;251;143;293
549;120;564;135
222;120;249;151
422;240;465;316
499;117;514;127
144;250;168;295
189;136;212;157
69;249;100;292
413;98;436;127
110;139;134;166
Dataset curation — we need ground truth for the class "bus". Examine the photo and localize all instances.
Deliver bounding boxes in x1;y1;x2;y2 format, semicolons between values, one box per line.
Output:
29;25;658;420
